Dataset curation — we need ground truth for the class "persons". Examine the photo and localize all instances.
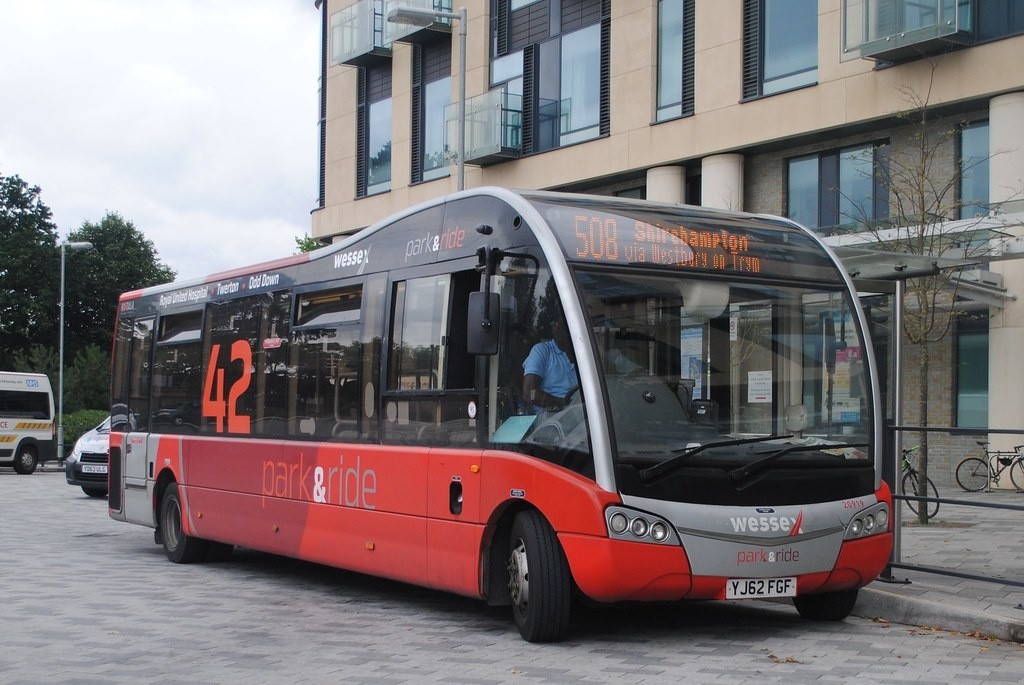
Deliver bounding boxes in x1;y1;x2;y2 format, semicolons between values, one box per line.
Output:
523;304;644;428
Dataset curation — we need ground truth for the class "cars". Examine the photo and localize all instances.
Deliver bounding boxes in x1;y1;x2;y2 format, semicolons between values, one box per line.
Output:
152;398;208;430
63;413;111;497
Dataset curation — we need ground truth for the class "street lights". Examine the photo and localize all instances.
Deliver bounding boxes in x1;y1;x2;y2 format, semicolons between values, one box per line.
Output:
55;241;95;467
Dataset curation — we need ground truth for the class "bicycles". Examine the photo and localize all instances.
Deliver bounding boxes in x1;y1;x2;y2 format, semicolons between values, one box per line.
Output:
955;439;1024;491
902;445;940;518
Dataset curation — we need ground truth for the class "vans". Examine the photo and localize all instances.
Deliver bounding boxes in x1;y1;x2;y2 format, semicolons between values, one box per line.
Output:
0;371;59;475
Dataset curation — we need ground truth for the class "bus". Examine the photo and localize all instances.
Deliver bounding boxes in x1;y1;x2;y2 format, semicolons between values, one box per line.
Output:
106;184;894;644
